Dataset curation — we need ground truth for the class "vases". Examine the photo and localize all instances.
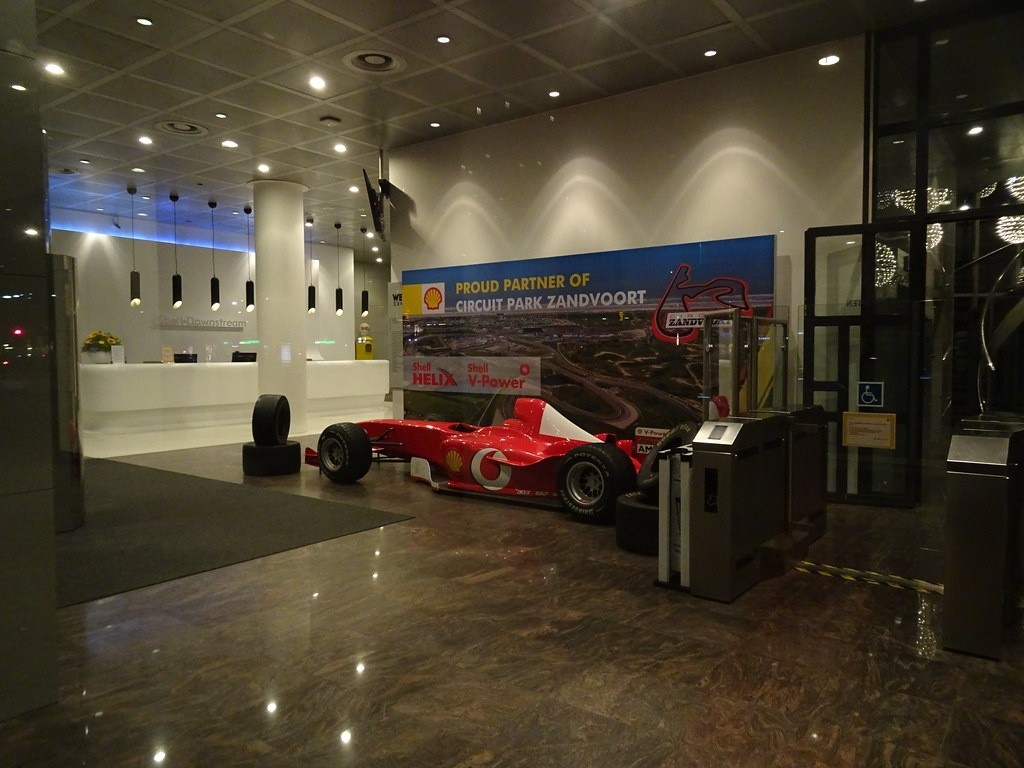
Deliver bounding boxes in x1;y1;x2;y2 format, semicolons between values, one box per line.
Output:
89;351;112;363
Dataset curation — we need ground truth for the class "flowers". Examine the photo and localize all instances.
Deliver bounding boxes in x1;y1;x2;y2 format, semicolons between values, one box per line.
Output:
82;330;120;353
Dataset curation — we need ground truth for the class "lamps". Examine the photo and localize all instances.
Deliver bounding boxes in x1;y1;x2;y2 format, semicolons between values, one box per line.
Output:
244;207;254;313
168;195;183;308
360;228;369;317
334;224;343;316
208;201;220;311
127;187;141;309
306;219;316;313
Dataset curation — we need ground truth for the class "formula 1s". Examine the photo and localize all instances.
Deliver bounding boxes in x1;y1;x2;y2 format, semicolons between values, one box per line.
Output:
304;397;647;521
